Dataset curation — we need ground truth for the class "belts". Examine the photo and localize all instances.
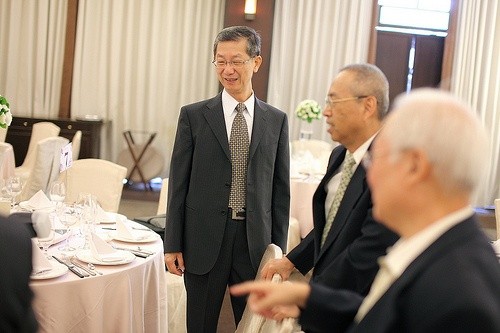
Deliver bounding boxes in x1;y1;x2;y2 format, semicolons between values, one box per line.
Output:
227;208;246;221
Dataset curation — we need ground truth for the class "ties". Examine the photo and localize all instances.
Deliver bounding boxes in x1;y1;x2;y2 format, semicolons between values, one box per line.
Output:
229;103;250;213
319;155;358;251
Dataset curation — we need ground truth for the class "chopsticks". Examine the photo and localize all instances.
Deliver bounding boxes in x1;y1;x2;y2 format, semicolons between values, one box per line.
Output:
51;255;84;278
131;252;147;258
101;227;151;231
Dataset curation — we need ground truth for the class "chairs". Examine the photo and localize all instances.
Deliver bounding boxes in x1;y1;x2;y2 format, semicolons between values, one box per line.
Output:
234;244;283;333
0;121;128;214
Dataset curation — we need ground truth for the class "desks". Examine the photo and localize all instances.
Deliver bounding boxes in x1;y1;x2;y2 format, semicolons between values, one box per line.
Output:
10;201;167;333
289;154;329;239
117;131;164;191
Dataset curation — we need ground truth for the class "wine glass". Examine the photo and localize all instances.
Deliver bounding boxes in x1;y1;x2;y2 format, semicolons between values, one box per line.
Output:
0;176;94;260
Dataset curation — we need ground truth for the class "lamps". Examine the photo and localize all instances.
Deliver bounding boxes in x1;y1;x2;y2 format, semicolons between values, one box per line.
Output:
243;0;257;20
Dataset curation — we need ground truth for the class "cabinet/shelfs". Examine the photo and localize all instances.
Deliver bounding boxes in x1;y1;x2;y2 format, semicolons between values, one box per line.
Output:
4;117;104;168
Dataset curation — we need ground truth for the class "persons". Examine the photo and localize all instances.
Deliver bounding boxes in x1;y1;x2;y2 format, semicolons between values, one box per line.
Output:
230;87;500;333
261;62;400;333
163;26;291;333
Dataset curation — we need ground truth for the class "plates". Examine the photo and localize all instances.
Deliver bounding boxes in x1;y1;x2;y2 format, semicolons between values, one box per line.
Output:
17;200;160;279
289;158;328;183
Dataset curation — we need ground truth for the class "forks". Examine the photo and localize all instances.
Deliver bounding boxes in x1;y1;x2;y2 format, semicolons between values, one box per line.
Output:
65;260;90;277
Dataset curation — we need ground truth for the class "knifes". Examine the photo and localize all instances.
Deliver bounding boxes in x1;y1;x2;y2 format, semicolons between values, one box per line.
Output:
70;259;96;276
114;246;154;255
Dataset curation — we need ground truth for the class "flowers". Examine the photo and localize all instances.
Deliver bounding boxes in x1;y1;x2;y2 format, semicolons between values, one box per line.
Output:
0;95;13;128
295;99;323;123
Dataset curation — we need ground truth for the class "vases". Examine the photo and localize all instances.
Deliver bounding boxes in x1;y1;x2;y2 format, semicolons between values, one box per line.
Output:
299;120;313;143
0;128;8;143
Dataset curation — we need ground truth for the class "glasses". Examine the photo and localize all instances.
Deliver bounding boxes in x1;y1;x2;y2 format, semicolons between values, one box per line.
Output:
212;56;256;68
323;95;377;108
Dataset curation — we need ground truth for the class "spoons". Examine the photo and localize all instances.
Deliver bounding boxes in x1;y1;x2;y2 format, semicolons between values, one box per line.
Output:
88;263;103;276
